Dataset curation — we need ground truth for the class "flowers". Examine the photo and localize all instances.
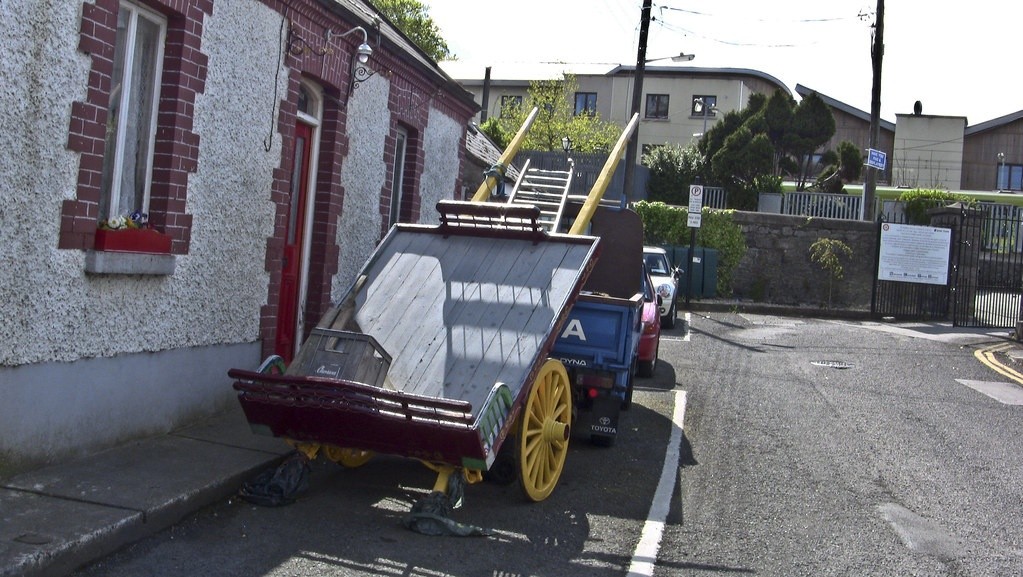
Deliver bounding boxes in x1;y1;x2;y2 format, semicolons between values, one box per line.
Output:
97;209;156;232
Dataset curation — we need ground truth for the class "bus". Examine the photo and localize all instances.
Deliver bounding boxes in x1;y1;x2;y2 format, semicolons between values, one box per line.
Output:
780;181;1023;288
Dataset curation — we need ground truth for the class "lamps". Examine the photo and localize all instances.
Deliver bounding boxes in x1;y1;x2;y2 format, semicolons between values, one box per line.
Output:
326;27;373;63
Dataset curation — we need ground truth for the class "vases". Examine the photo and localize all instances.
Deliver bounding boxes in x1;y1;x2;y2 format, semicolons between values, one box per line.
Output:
95;228;173;253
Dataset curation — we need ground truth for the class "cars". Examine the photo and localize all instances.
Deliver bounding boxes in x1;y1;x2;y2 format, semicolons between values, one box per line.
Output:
642;246;684;328
629;264;664;377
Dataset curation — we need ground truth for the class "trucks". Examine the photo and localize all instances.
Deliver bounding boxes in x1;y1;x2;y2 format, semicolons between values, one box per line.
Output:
488;197;648;446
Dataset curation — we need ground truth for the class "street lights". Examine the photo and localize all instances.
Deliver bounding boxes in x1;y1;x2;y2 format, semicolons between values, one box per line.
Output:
620;53;697;210
561;134;573;171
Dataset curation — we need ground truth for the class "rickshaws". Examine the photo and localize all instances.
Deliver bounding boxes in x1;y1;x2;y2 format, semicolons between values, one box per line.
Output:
229;106;641;527
